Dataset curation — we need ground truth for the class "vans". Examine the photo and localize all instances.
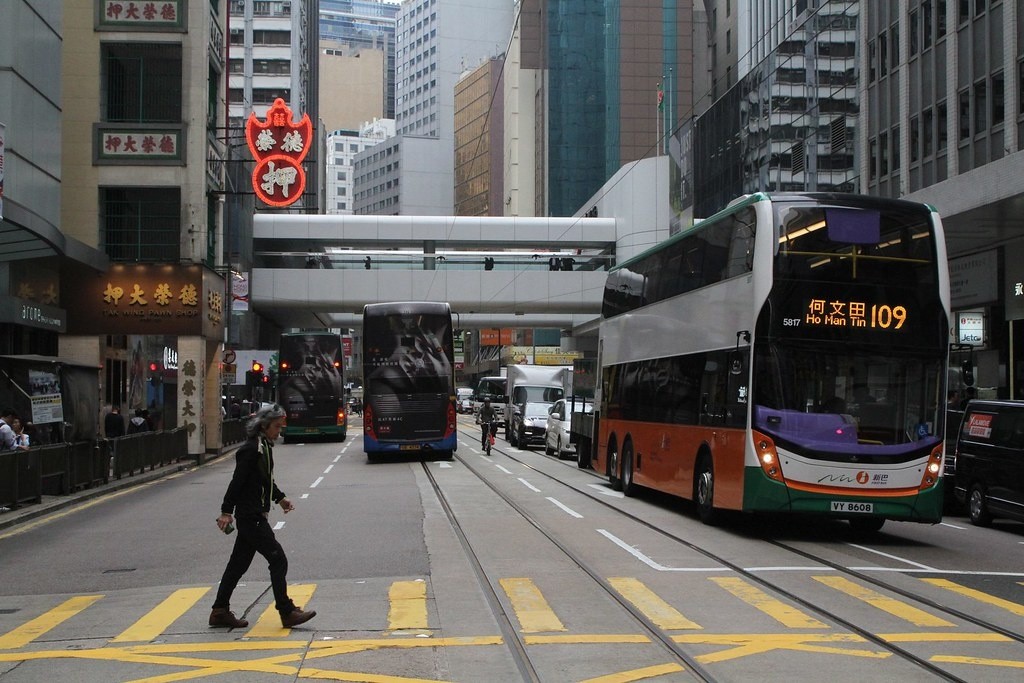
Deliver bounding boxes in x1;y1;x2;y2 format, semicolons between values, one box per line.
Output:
955;397;1024;530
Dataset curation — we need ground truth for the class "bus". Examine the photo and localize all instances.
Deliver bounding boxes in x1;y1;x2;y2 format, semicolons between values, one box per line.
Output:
571;186;949;534
363;301;459;464
278;331;348;444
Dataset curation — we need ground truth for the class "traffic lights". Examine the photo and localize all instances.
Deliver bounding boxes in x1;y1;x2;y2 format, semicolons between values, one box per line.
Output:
263;375;269;385
253;363;264;387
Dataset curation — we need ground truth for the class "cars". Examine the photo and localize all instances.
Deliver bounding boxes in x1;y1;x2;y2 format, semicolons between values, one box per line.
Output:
543;396;596;461
460;400;473;414
512;402;557;450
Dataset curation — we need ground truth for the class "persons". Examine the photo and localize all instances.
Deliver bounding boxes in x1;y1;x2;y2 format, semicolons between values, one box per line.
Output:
0;405;35;514
476;398;499;451
343;394;363;418
126;407;147;437
143;410;155;433
104;404;126;465
208;405;318;628
219;395;269;422
946;389;960;441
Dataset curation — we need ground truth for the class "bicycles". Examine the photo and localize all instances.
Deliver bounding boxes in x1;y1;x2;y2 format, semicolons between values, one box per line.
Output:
476;418;500;456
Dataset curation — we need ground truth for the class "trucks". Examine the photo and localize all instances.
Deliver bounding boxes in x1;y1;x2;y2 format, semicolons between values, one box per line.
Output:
505;362;574;442
455;387;474;412
474;376;506;429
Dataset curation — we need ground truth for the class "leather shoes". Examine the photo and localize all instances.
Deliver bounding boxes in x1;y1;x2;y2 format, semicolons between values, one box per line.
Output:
281;603;316;629
209;607;249;627
